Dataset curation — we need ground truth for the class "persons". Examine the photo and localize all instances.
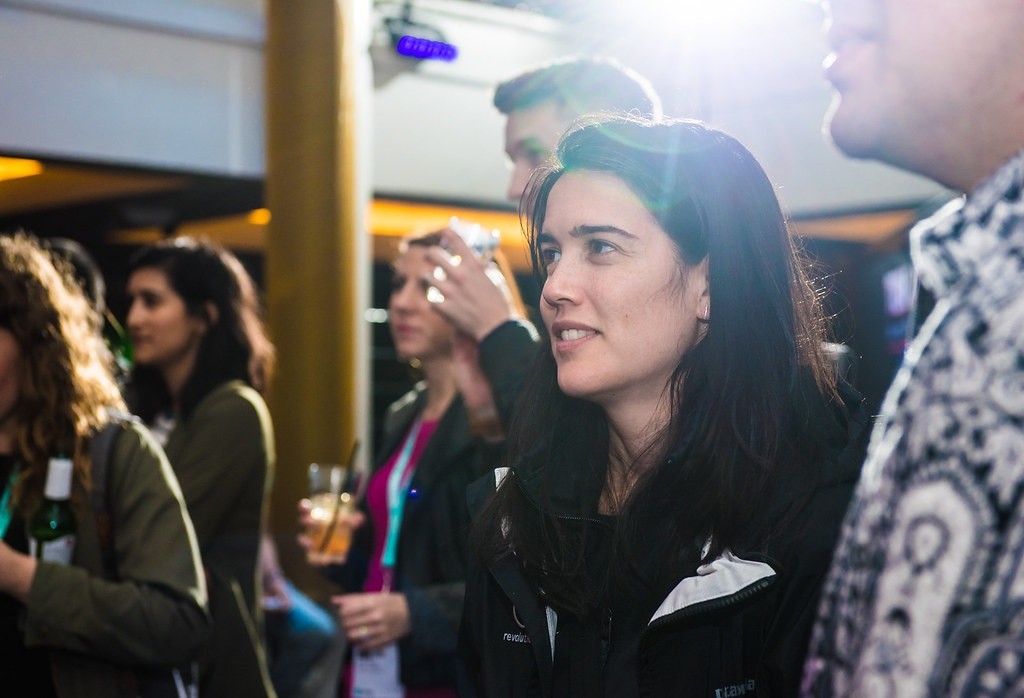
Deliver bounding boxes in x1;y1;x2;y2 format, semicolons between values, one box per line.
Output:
0;0;1024;697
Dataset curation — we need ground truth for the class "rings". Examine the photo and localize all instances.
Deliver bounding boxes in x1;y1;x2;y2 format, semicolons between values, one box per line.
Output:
357;627;369;640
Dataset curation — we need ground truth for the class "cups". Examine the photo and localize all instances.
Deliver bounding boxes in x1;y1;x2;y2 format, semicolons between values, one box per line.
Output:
427;217;501;303
304;464;364;563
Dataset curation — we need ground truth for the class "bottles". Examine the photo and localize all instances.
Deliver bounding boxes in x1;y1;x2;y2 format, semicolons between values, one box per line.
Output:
25;451;81;564
